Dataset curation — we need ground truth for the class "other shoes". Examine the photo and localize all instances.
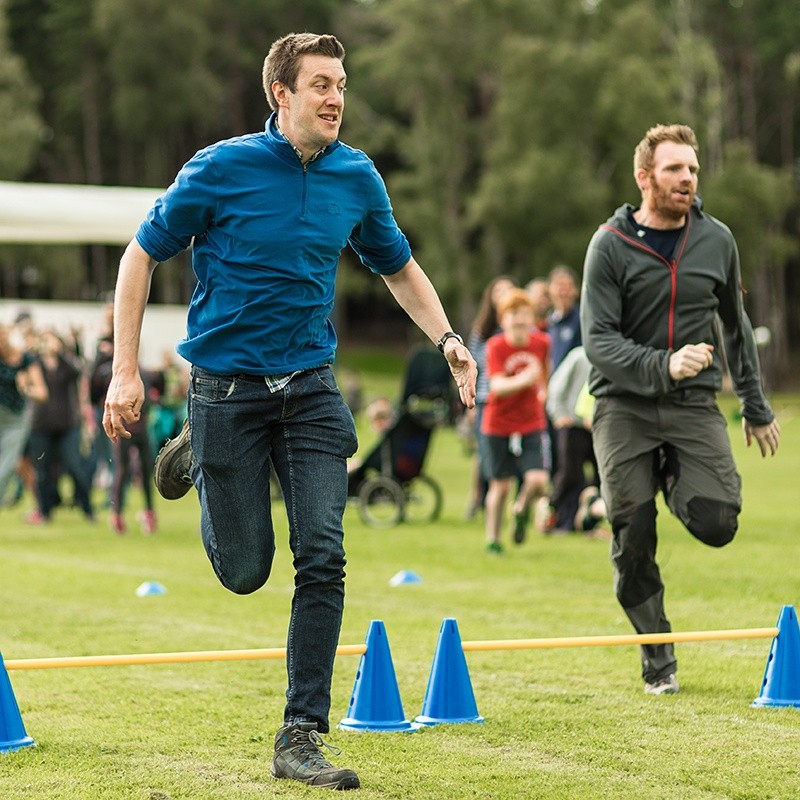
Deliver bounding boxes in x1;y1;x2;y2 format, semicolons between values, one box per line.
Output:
573;485;601;531
25;511;51;525
141;509;156;532
111;516;125;535
490;543;502;553
514;509;528;543
537;495;559;537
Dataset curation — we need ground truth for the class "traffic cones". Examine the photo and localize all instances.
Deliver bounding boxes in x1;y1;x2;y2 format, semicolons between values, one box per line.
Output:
338;618;425;735
0;653;36;752
414;618;485;727
752;604;800;709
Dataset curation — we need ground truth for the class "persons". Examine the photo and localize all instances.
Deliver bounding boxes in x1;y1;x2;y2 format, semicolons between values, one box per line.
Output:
578;122;783;696
102;29;478;790
0;267;607;557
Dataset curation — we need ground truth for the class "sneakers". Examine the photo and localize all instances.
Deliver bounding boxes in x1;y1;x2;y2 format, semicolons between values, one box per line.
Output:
153;417;197;500
643;672;678;697
271;722;360;790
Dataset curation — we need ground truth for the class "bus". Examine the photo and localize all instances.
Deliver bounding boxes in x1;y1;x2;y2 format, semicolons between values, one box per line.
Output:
0;180;201;398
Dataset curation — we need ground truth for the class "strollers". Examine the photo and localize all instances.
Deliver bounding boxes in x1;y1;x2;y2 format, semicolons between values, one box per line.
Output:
345;346;453;530
